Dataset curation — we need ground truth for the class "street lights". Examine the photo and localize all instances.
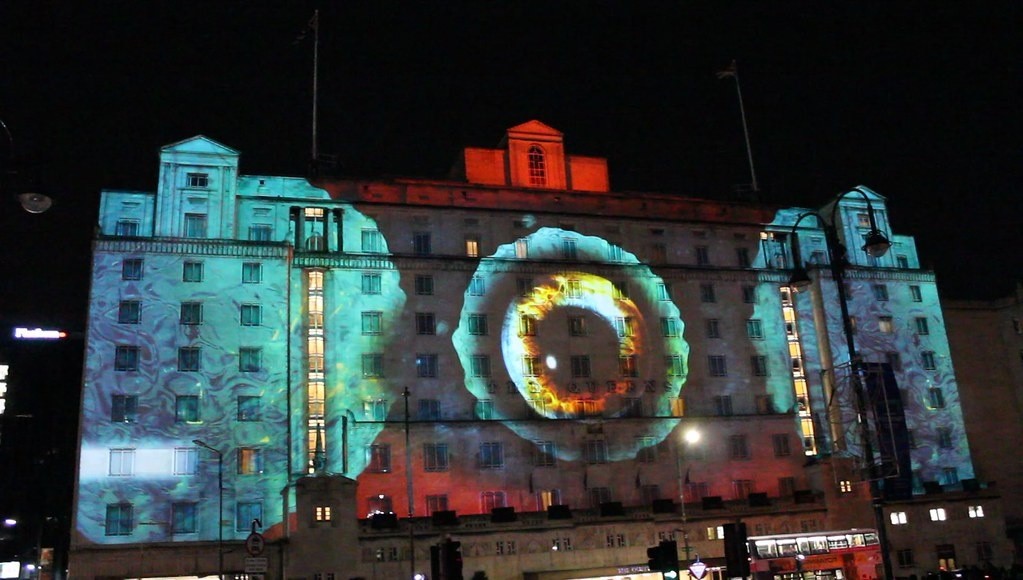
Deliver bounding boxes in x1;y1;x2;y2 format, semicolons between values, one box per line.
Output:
675;425;702;580
192;440;223;580
786;187;898;580
402;385;415;580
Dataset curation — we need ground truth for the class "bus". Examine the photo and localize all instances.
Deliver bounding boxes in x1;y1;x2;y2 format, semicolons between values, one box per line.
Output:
746;528;888;579
